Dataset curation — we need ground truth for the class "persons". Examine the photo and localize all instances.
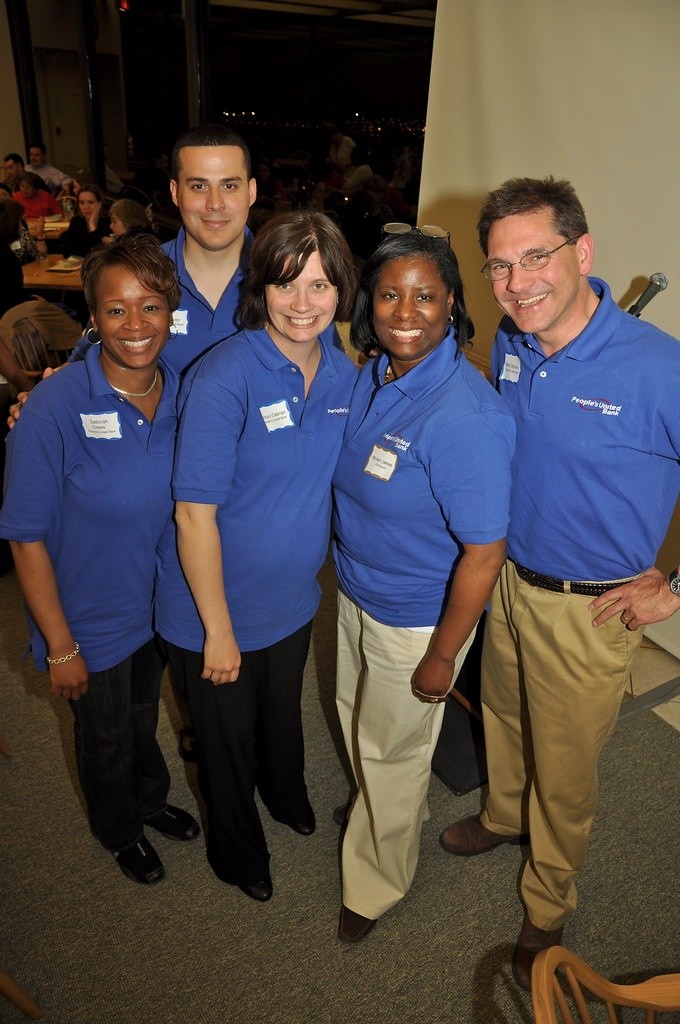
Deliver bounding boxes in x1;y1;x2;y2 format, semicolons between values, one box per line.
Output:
154;210;378;903
8;122;346;762
476;177;680;990
334;222;516;943
0;125;420;404
0;231;201;885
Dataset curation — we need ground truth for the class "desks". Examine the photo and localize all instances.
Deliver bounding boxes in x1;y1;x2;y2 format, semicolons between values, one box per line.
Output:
21;217;69;240
21;254;84;294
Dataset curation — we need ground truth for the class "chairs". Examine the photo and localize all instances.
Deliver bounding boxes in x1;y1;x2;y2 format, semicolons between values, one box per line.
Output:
528;947;679;1024
55;196;77;218
0;301;82;384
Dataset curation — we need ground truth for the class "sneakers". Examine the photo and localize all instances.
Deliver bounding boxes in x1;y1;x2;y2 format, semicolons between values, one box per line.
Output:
144;804;201;842
112;837;165;884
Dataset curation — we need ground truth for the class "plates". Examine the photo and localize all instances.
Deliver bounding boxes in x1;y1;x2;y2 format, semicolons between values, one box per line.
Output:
46;262;81;271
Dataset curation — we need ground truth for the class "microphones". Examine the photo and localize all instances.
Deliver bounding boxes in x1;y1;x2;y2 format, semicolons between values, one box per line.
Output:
626;272;669;318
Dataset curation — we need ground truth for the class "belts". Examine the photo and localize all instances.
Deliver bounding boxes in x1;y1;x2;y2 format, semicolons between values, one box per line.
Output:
504;554;636;596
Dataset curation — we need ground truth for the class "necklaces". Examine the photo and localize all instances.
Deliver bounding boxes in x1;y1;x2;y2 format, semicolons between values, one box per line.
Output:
384;365;392;384
107;368;158;396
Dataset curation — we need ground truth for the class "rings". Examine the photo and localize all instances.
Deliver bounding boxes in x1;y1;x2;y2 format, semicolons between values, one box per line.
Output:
623;613;631;621
430;698;439;702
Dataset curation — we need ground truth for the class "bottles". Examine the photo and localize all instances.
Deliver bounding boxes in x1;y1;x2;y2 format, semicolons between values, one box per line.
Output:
106;233;118;246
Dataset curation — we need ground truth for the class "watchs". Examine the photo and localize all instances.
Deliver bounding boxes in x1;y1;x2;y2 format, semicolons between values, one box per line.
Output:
668;568;680;596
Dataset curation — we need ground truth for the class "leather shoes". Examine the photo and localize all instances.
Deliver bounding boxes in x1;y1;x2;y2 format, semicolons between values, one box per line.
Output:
440;814;532;855
239;879;273;902
513;913;568;989
289;805;316;836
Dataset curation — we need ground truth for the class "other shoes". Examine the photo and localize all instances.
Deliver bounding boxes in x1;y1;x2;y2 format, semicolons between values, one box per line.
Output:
337;905;378;941
333;804;352;823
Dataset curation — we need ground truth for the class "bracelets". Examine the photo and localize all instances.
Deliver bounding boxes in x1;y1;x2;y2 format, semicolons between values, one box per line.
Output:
47;641;80;664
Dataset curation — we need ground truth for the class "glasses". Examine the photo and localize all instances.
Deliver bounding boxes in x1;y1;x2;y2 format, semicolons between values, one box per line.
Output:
480;230;589;283
377;222;452;251
